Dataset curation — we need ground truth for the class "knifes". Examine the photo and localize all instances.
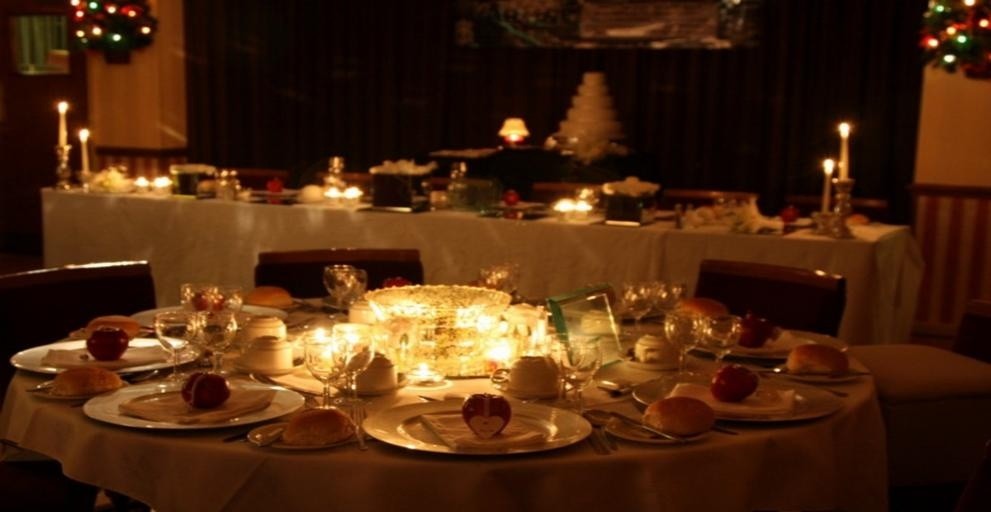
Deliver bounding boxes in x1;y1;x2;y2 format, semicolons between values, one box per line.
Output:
610;410;687;441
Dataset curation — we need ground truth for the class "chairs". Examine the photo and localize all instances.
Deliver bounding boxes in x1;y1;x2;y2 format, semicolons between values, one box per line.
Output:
695;255;849;338
2;258;157;510
252;246;426;298
903;184;991;357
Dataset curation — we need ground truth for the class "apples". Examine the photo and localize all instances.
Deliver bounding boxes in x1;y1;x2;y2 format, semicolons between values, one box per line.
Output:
732;316;770;348
462;393;512;440
585;280;617;307
192;290;225;316
710;365;759;403
87;326;129;361
182;373;231;409
384;278;411;287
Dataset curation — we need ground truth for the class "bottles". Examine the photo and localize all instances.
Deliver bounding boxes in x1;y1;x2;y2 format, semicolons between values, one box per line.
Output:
173;155;817;235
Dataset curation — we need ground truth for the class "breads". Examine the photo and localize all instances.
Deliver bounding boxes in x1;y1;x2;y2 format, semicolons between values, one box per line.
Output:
642;396;717;436
84;315;141;340
785;345;849;376
469;280;517;303
243;286;293;310
52;367;123;396
283;406;354;445
675;297;729;321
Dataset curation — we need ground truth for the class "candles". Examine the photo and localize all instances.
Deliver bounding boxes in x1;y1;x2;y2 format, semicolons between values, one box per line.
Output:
838;122;852;182
57;99;69;148
76;127;91;175
821;158;837;215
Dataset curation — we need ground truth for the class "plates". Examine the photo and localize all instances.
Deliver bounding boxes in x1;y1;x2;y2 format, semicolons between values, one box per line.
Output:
629;370;842;423
698;331;850;356
33;377;130;402
13;339;200;374
605;414;714;443
771;362;862;382
84;379;306;435
364;400;595;454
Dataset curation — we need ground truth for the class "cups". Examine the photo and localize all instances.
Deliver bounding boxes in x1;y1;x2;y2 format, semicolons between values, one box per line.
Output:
476;259;683;397
668;309;743;370
493;355;562;397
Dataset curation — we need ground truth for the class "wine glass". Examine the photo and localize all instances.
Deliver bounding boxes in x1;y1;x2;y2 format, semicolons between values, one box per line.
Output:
153;264;375;424
552;333;602;425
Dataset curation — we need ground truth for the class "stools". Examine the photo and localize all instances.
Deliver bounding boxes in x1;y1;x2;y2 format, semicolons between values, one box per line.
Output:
847;338;991;509
